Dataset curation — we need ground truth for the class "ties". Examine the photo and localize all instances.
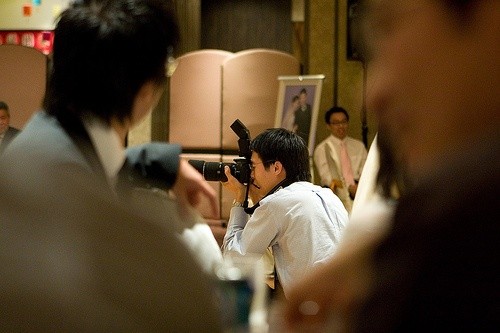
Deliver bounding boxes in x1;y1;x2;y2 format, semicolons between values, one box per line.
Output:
340;144;355;187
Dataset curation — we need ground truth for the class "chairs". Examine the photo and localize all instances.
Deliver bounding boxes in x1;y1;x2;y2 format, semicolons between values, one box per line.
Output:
131;186;222;275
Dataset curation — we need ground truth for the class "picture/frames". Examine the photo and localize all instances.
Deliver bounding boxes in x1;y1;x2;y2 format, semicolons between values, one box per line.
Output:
274;75;326;156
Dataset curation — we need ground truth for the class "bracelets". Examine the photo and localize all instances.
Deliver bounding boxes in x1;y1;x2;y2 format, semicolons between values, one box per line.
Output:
232;199;245;208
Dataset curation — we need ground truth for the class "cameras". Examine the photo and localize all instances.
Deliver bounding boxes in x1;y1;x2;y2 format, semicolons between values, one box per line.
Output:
187;119;252;186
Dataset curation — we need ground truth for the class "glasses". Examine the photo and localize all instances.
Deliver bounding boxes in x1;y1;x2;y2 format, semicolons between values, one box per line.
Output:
248;159;275;169
164;56;180;78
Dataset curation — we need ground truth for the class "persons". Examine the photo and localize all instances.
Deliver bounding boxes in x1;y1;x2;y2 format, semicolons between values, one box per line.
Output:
221;130;349;297
294;88;312;146
282;96;300;133
0;0;500;333
